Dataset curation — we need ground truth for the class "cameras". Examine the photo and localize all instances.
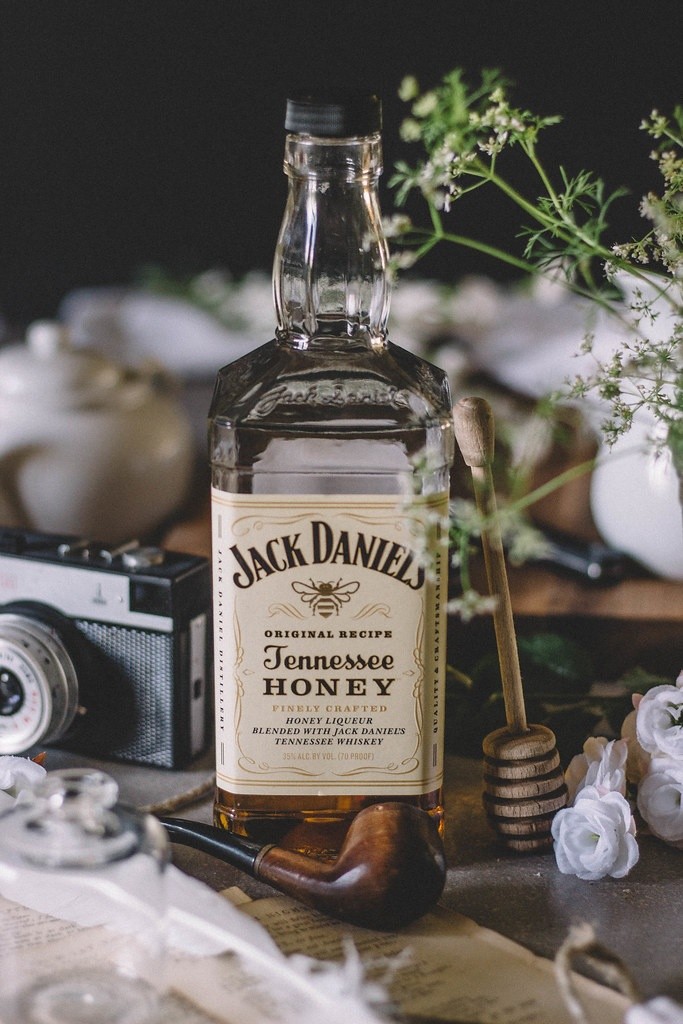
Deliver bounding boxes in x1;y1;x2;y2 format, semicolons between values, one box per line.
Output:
0;526;210;773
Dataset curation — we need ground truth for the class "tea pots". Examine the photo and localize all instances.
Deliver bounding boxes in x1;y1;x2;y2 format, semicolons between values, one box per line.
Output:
1;318;200;546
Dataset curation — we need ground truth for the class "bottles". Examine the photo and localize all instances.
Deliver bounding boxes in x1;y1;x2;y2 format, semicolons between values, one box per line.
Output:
206;84;457;869
0;764;172;1024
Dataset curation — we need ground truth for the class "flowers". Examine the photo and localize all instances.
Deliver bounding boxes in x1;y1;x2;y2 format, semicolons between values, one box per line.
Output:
367;62;682;729
549;675;682;881
